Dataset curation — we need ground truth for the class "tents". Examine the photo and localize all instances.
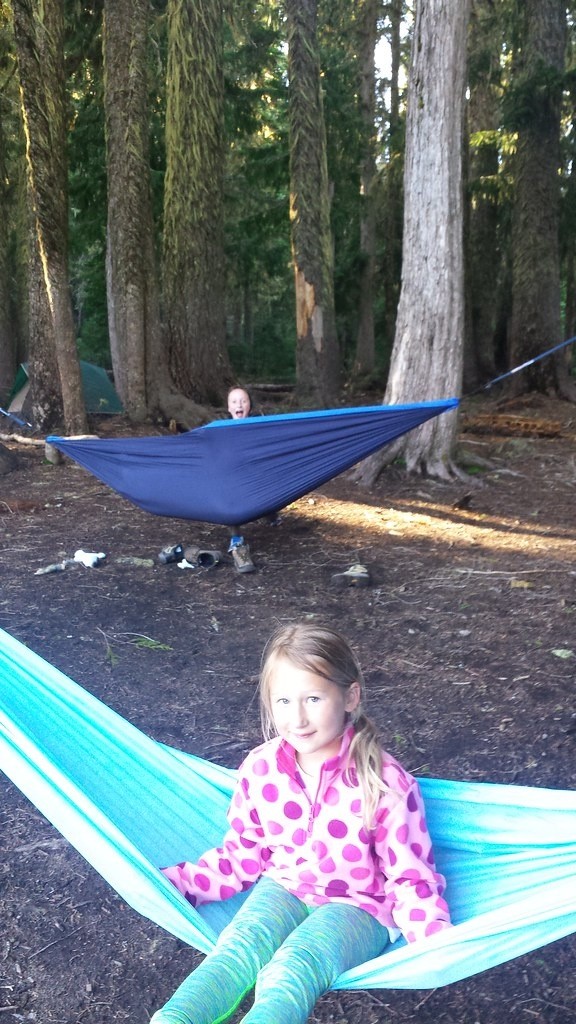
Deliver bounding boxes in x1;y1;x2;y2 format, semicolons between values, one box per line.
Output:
3;359;127;418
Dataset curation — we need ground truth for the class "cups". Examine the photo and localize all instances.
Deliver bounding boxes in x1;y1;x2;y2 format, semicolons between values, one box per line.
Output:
197;551;219;569
158;544;183;563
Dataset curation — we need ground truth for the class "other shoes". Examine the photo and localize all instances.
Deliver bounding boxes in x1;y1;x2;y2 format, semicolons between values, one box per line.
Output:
183;549;221;567
332;565;370;588
232;545;254;575
159;545;182;565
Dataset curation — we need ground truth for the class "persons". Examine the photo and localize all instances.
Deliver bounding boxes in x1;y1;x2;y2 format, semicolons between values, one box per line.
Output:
203;385;283;574
149;622;455;1024
